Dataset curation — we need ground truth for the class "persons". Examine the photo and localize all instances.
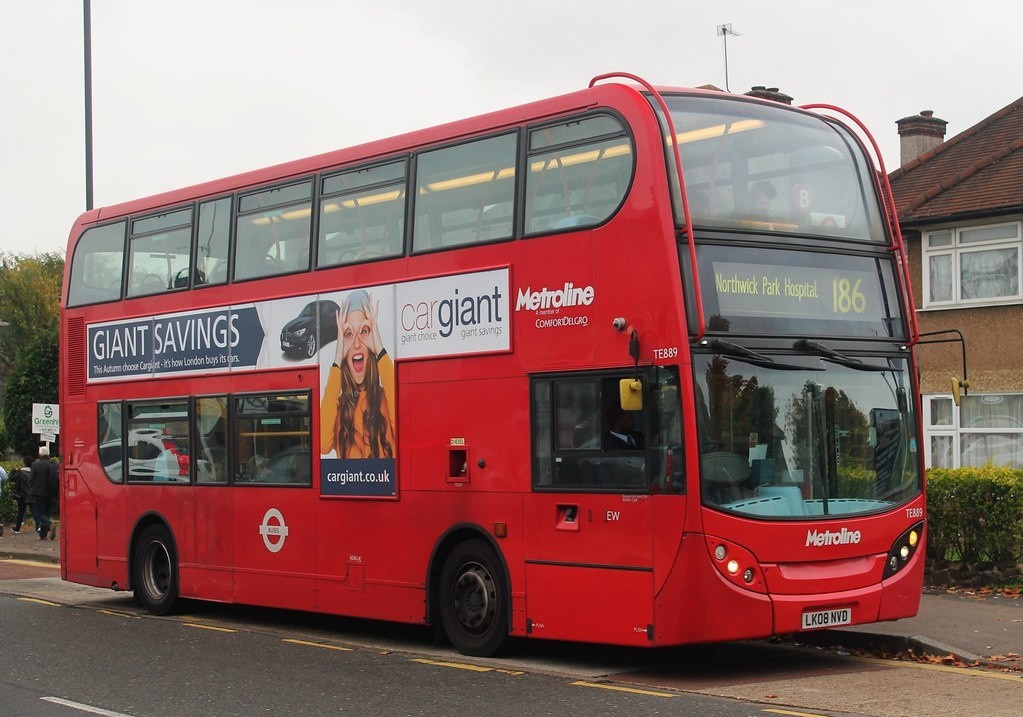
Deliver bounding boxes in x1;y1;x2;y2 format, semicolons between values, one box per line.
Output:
0;445;59;540
601;400;670;488
320;288;395;459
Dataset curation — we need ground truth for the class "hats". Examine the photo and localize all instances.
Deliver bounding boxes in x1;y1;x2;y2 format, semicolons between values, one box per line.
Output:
337;290;374;319
37;446;49;456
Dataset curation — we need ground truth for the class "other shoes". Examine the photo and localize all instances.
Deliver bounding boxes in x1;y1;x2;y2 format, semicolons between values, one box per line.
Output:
38;523;56;541
10;524;20;533
34;522;41;533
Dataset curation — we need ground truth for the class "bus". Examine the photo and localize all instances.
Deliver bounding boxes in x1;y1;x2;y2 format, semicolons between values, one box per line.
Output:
56;72;969;662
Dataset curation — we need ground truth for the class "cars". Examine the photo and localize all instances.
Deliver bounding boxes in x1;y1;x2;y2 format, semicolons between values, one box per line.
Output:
98;438;122;483
129;426;216;482
